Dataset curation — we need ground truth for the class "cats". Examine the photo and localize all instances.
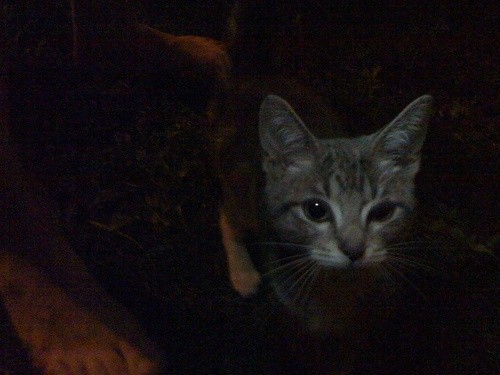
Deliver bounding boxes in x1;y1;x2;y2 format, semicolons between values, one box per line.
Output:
203;0;439;336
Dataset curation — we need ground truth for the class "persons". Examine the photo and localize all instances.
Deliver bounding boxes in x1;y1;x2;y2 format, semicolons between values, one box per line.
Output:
1;0;171;375
